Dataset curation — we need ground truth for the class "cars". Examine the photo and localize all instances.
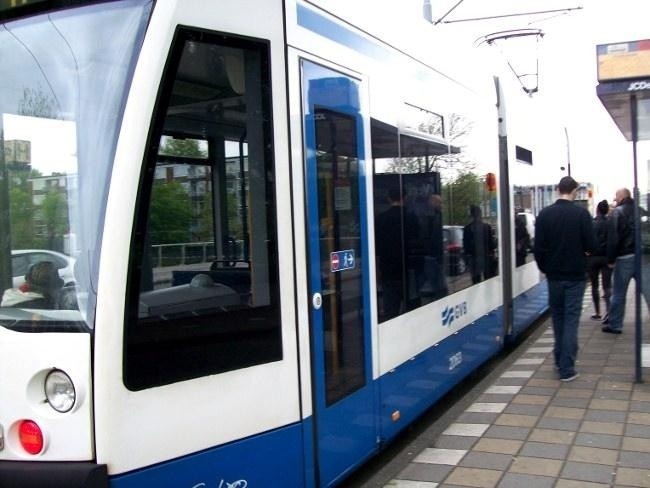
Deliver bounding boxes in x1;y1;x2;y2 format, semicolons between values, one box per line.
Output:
2;246;81;306
441;220;468;277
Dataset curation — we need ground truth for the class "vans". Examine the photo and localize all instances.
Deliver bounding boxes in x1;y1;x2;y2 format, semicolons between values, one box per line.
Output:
518;208;537;255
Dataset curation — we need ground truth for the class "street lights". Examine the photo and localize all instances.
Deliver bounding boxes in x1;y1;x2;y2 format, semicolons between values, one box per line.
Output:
447;126;476;223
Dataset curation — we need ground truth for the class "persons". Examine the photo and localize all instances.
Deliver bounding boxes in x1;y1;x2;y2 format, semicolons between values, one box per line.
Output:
514;208;530;267
600;187;635;334
417;193;448;299
589;199;610;320
375;182;423;322
531;174;596;383
461;203;497;285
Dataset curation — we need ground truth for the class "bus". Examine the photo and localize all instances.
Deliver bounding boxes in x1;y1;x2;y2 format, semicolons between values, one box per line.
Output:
0;0;606;488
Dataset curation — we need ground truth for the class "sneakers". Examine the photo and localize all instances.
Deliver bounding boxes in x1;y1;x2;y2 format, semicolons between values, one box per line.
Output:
555;361;579;382
592;313;622;334
